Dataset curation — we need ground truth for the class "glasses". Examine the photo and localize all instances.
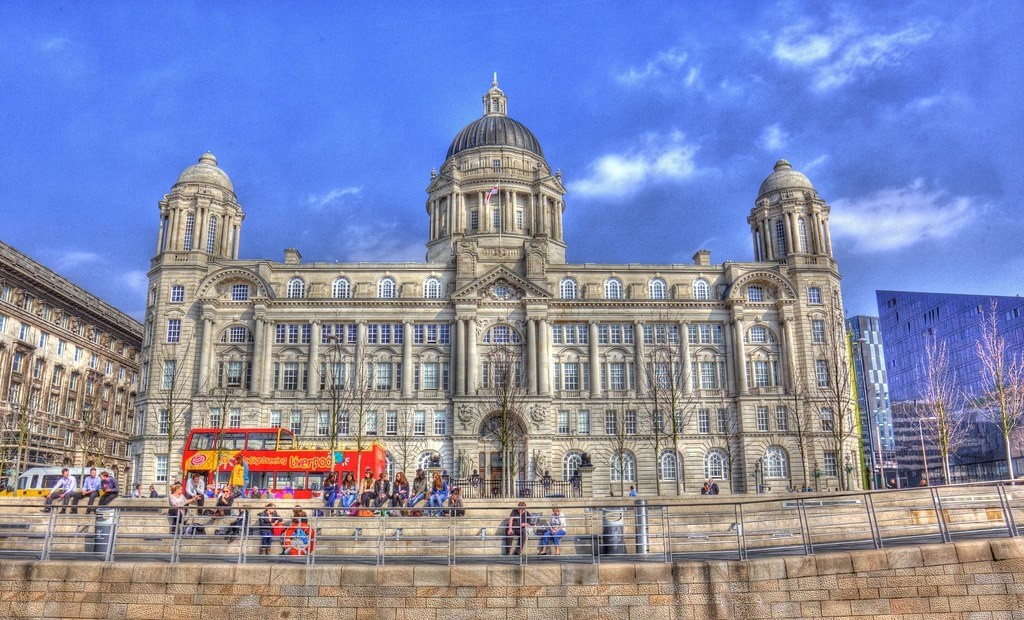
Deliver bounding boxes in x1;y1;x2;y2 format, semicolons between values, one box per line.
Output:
223;491;228;493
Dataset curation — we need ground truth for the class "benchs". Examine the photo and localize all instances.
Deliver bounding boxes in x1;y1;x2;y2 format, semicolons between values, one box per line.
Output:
0;499;450;516
0;526;793;554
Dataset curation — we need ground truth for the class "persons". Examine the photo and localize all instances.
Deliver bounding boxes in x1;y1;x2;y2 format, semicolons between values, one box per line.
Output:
133;484;273;499
886;477;897;489
538;504;566;555
629;484;637;496
792;484;814;493
70;468;102;514
169;473;250;541
701;477;719;494
287;505;307;543
230;455;249;498
505;501;531;556
536;450;545;477
90;471;120;512
258;504;283;555
919;479;928;486
459;449;470;478
40;468;77;514
323;468;465;516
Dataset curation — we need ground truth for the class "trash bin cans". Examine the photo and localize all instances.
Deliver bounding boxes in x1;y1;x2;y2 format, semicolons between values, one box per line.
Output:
94;508;119;560
602;508;623;554
311;490;324;499
759;484;772;493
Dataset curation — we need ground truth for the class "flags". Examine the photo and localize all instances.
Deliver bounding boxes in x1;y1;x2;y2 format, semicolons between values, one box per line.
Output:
485;183;498;202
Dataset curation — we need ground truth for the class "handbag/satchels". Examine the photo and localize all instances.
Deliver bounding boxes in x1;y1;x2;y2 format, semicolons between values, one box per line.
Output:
358;509;373;517
530;512;542;525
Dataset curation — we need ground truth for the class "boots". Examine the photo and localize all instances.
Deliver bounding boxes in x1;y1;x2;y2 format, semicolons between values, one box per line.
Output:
261;548;272;555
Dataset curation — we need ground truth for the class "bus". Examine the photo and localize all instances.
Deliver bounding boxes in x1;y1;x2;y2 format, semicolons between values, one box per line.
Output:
178;427;388;500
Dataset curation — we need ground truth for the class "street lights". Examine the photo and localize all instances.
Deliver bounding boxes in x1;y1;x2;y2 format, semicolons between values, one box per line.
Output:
917;416;938;486
814;459;819;492
755;460;759;494
845;453;851;491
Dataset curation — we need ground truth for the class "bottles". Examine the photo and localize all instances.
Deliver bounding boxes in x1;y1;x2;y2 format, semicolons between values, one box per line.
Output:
382;510;384;516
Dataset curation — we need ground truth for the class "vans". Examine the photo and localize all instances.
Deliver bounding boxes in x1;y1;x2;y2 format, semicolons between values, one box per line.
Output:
0;467;114;497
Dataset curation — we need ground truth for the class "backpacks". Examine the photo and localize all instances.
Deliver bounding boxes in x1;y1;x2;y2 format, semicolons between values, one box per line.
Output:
350;502;360;516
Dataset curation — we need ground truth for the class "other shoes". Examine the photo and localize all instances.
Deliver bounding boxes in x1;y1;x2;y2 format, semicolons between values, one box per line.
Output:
555;549;560;555
515;550;520;555
538;549;546;555
40;507;98;515
506;551;510;555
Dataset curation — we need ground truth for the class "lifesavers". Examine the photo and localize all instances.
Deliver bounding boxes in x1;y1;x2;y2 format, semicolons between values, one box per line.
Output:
284;523;317;556
